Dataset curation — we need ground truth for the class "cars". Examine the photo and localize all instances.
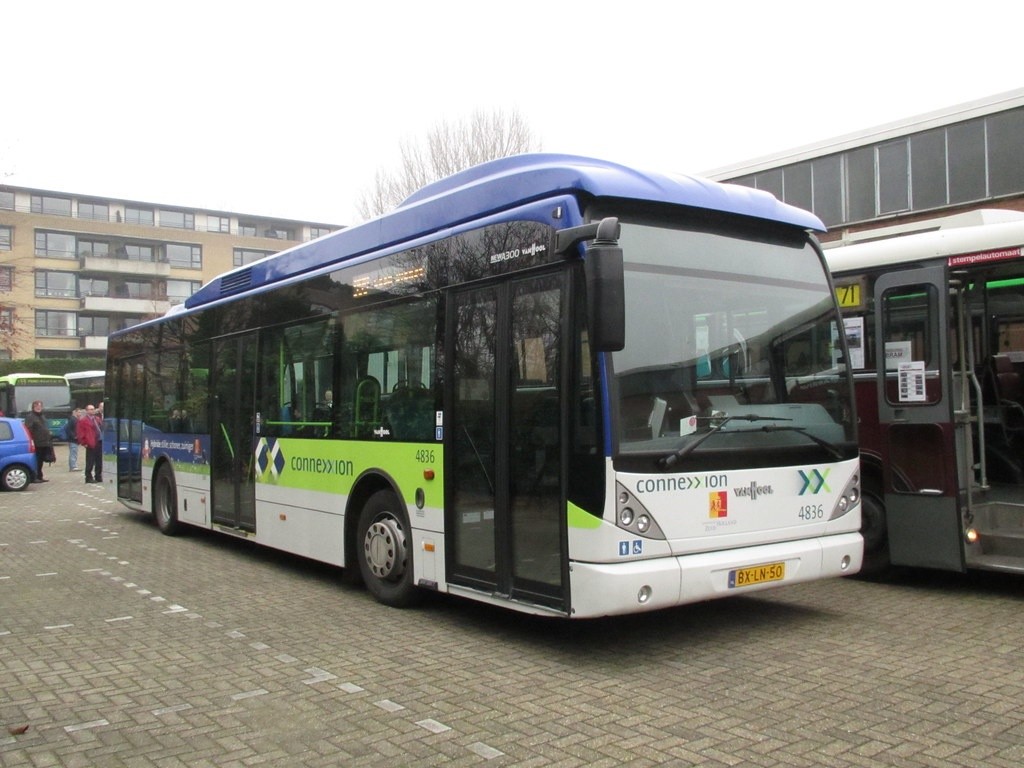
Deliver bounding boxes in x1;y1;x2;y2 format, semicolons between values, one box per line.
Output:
0;417;39;491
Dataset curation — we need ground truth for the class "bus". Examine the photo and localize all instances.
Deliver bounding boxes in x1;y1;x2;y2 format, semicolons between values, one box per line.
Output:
102;150;865;625
0;373;73;440
63;371;105;419
786;206;1023;580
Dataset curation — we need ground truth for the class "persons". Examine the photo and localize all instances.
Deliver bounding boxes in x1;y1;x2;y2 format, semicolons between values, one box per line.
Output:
325;391;333;410
24;401;50;483
77;404;103;483
97;403;104;425
65;408;82;471
171;409;192;434
